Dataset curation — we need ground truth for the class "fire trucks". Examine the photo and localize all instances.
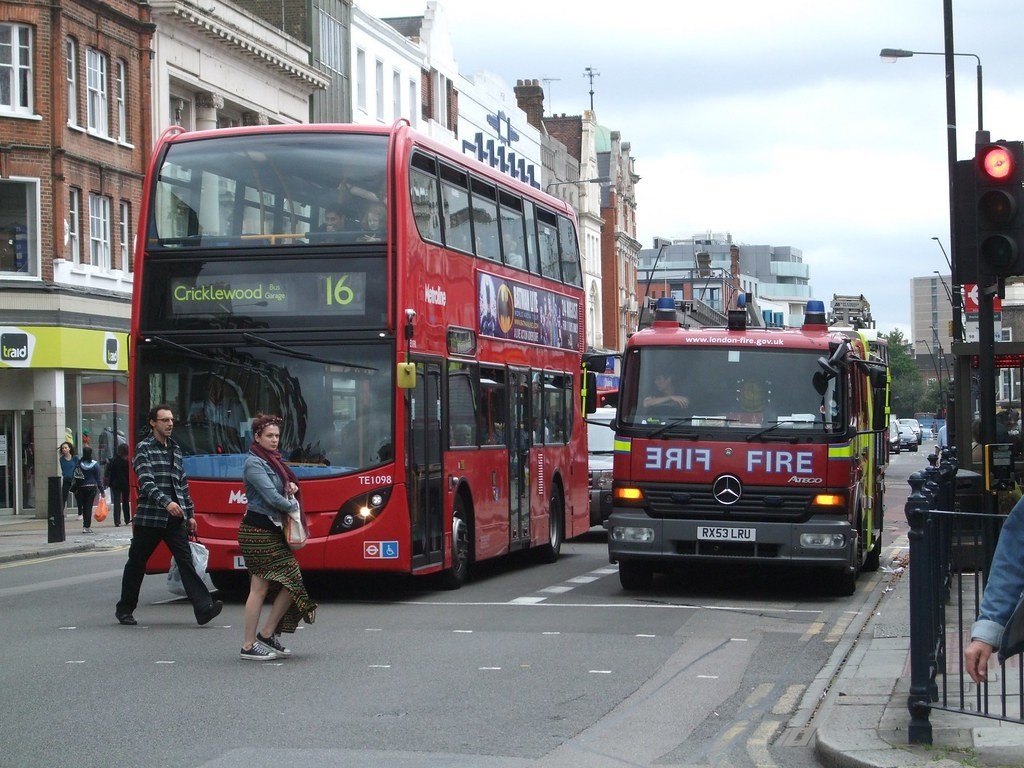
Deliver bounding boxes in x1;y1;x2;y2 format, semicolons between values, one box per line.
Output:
580;296;891;597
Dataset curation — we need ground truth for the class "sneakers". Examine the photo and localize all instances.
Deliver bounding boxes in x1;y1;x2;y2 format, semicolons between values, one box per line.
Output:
82;527;93;533
197;599;223;625
240;641;277;661
256;631;293;658
115;609;137;625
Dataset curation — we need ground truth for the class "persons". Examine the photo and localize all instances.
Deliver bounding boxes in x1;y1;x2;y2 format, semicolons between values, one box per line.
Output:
97;427;127;464
102;443;134;527
235;412;321;661
72;446;105;533
965;494;1024;683
64;426;74;445
453;405;555;445
82;428;92;448
56;442;83;519
503;232;524;268
312;201;360;242
642;364;694;408
114;404;223;626
355;204;387;243
934;407;1020;468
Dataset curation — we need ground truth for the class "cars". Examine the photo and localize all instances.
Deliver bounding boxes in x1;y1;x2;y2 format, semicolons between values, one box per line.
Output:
888;418;923;454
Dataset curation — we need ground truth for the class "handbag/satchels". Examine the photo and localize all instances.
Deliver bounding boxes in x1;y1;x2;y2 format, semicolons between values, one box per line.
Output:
69;461;85;493
95;495;108;522
167;529;218;595
283;489;308;550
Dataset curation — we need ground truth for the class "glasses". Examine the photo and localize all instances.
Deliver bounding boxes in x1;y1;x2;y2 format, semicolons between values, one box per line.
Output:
156;418;175;422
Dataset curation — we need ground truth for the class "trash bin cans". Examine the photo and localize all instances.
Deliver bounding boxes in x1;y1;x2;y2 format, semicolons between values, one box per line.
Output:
954;468;984;539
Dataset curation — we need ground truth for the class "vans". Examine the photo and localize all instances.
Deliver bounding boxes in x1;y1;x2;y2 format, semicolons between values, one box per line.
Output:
584;407;617;527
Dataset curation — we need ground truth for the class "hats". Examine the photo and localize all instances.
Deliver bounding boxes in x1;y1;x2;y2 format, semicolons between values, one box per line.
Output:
104;427;113;433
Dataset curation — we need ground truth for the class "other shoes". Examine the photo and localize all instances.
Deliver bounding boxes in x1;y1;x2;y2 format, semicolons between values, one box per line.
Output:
76;515;83;520
125;520;130;524
116;521;121;527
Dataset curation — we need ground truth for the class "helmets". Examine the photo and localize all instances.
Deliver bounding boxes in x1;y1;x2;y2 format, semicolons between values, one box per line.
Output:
738;378;769;412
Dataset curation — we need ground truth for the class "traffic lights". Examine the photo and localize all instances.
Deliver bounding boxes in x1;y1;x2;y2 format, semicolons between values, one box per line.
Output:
972;140;1023;275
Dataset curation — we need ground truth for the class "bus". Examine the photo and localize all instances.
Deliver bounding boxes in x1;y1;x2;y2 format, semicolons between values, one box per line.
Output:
125;117;589;596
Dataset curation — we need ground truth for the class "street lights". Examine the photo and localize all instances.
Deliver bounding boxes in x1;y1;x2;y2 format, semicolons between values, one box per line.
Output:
934;270;966;341
700;275;713;300
915;340;941;409
638;243;670;330
931;236;966;309
929;326;950;381
878;48;996;466
723;288;738;316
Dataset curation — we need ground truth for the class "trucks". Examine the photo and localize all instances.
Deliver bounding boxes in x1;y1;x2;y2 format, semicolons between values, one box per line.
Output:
913;412;936;440
